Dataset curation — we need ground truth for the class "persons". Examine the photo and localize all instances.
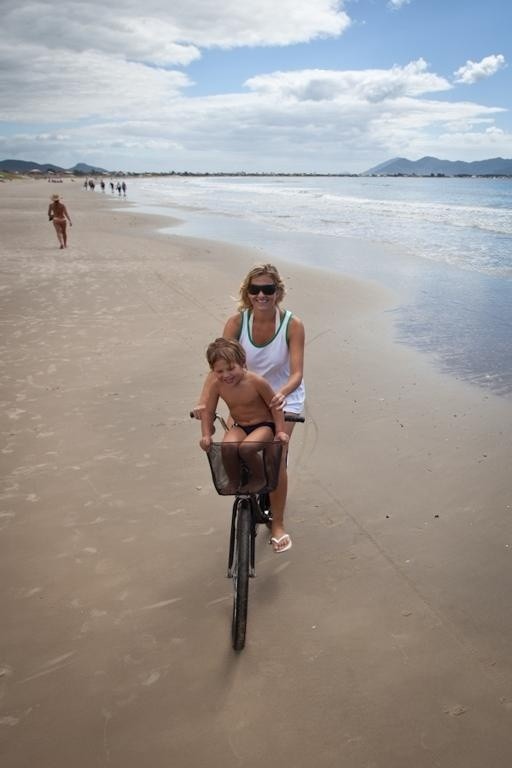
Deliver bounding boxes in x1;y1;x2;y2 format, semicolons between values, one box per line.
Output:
198;336;290;496
47;192;73;249
81;179;128;196
189;260;306;554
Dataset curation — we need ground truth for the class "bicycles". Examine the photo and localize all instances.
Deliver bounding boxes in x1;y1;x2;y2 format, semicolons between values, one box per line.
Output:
188;406;304;652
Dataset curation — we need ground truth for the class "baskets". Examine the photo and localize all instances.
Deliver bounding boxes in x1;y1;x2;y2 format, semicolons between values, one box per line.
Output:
207;440;283;495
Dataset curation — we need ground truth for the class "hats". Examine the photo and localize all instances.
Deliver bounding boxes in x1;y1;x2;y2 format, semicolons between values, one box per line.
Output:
49;192;63;200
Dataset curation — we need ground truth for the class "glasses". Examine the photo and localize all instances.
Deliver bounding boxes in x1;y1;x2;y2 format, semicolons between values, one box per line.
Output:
248;283;276;294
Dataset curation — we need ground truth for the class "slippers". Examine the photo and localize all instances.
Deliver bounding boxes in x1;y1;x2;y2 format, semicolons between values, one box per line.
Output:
270;533;292;552
